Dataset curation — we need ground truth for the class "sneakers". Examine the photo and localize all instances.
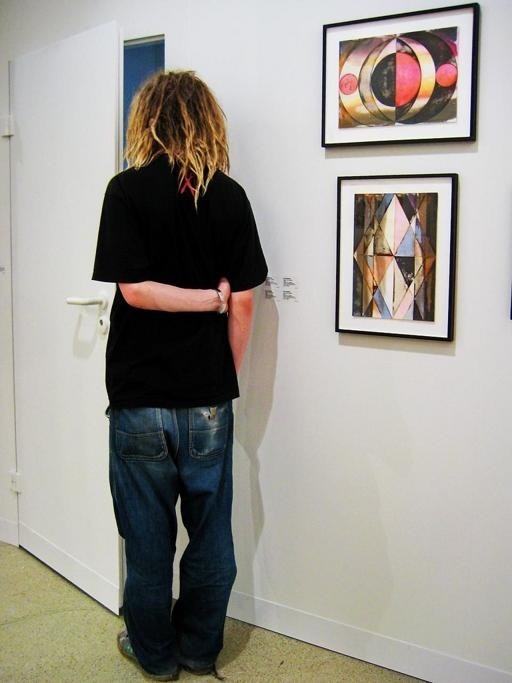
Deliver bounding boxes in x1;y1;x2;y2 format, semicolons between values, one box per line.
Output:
117;629;215;682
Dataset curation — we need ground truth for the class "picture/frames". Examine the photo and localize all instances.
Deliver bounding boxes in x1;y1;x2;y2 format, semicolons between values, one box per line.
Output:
322;2;480;147
336;173;458;342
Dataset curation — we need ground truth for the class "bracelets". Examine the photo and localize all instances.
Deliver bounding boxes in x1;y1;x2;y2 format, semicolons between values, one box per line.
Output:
215;290;226;314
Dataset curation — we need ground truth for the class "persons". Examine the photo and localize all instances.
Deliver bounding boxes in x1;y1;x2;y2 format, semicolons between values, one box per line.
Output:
91;70;269;682
